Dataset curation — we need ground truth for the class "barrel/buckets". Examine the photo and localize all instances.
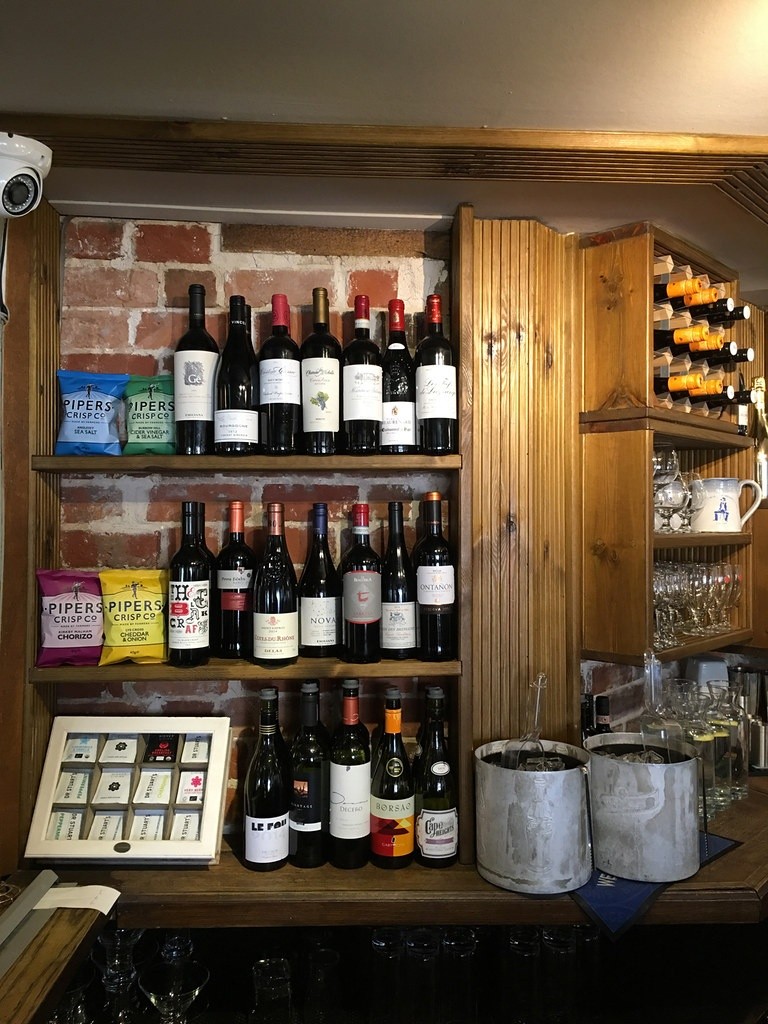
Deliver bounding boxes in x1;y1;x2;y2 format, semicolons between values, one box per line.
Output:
728;667;768;769
583;732;700;882
475;739;591;894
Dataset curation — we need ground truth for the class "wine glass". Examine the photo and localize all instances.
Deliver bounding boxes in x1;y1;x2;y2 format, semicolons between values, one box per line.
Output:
654;442;707;533
653;561;743;651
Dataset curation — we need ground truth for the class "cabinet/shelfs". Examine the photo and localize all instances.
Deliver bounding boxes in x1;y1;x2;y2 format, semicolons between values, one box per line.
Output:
579;220;757;667
0;205;475;869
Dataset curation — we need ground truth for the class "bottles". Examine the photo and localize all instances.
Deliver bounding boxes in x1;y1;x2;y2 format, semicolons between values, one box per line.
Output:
215;501;298;667
664;679;749;823
382;295;458;456
216;294;300;457
168;502;215;668
300;287;382;458
381;492;457;663
243;680;460;871
654;278;759;435
581;694;614;749
298;503;340;658
174;284;221;457
338;504;381;664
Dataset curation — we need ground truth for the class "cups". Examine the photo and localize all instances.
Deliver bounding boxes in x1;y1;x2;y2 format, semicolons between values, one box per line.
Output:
691;478;763;532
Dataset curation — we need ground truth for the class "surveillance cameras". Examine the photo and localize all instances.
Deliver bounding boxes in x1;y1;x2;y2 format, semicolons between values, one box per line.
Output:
0;131;53;217
